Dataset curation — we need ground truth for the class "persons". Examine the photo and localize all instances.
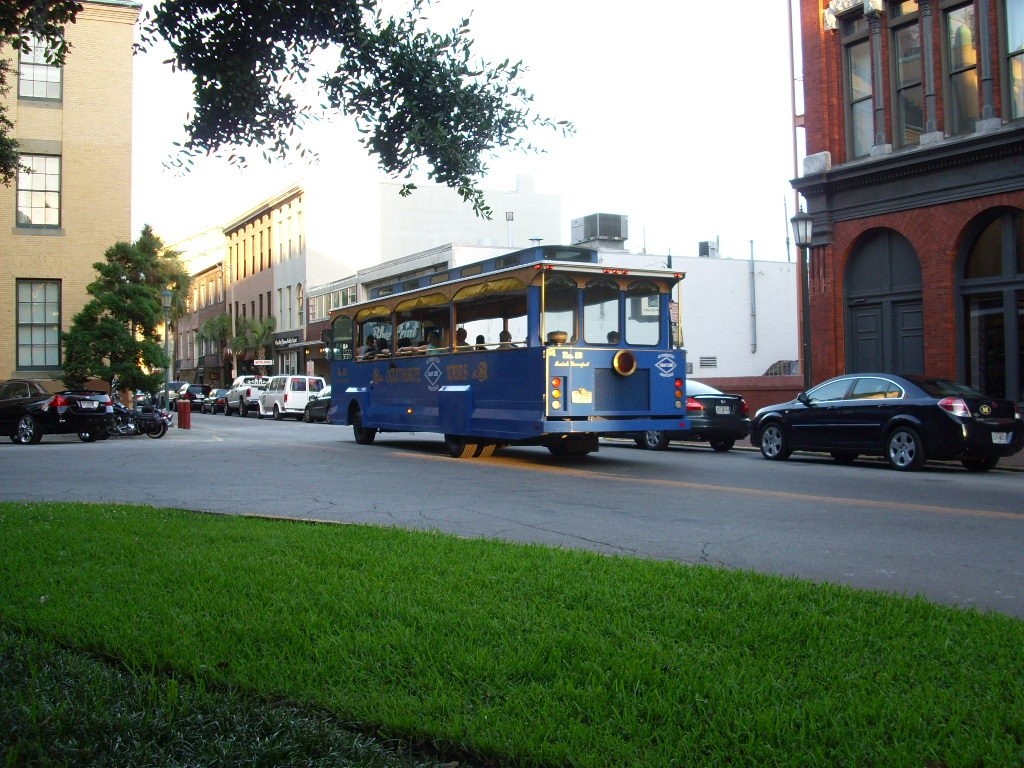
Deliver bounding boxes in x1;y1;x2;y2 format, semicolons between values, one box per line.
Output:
456;328;473;350
364;338;388;360
361;335;376;355
607;331;620;344
418;341;427;347
496;330;515;348
398;337;412;348
476;335;487;350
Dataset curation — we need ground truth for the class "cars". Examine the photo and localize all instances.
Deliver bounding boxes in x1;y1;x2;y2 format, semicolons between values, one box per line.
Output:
201;389;229;415
644;379;751;452
750;374;1024;473
304;385;366;423
0;379;113;445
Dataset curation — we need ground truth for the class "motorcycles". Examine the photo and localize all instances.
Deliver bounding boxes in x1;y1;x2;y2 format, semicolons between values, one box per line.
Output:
110;393;173;439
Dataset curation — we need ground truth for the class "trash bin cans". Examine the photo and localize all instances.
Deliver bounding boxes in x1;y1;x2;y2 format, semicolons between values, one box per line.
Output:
177;400;190;429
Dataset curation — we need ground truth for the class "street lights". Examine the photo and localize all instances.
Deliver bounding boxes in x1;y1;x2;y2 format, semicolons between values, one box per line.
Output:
790;205;817;390
160;292;174;411
203;253;237;381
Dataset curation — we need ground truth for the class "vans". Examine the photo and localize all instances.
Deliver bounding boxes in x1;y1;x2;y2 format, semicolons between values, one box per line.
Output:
256;376;327;421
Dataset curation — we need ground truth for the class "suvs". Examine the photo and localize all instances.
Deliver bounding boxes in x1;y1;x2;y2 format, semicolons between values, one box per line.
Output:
134;381;212;412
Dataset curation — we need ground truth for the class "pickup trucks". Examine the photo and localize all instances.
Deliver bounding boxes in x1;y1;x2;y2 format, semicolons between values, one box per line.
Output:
224;376;271;417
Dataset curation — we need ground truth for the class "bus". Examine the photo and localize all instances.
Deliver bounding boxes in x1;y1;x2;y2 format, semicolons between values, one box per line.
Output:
321;260;692;458
